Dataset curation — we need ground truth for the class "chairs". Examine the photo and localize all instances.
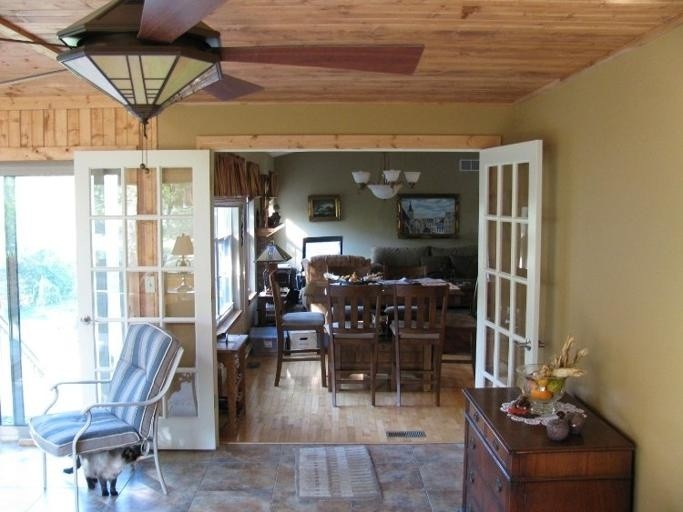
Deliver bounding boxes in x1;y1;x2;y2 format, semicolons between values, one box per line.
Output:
26;320;185;511
268;255;477;408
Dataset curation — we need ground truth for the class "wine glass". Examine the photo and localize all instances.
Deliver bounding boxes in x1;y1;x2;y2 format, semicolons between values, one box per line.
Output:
516;363;569;414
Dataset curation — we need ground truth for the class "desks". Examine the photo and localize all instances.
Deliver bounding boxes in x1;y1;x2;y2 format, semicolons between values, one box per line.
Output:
217;333;248;435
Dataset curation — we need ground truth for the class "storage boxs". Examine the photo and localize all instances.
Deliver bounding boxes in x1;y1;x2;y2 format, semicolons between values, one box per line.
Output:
249;326;288;358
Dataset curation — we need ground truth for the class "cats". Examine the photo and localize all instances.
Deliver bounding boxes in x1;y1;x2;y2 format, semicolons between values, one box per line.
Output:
62;444;146;497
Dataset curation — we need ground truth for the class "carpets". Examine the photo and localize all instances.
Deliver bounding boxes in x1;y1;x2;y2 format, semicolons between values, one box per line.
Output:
295;444;382;502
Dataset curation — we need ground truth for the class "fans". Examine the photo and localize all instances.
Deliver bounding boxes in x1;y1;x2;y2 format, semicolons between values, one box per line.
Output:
0;0;433;126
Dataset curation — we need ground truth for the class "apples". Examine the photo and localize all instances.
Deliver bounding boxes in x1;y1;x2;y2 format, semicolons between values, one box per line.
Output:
546;380;564;396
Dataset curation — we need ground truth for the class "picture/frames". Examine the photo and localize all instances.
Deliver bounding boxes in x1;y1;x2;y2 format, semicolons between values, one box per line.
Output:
398;193;460;240
308;194;342;222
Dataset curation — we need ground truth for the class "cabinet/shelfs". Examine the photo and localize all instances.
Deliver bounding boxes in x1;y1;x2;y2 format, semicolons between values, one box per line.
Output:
460;384;635;511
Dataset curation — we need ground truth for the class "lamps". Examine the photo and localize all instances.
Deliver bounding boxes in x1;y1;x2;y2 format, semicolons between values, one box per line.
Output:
56;0;223;178
350;152;423;203
171;232;194;292
256;245;292;295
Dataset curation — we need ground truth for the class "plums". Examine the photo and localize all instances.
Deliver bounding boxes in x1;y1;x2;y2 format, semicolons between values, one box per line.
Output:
563;412;585;435
546;419;569;441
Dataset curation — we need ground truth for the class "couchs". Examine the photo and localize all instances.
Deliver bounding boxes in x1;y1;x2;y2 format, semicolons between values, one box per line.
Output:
375;245;478;311
